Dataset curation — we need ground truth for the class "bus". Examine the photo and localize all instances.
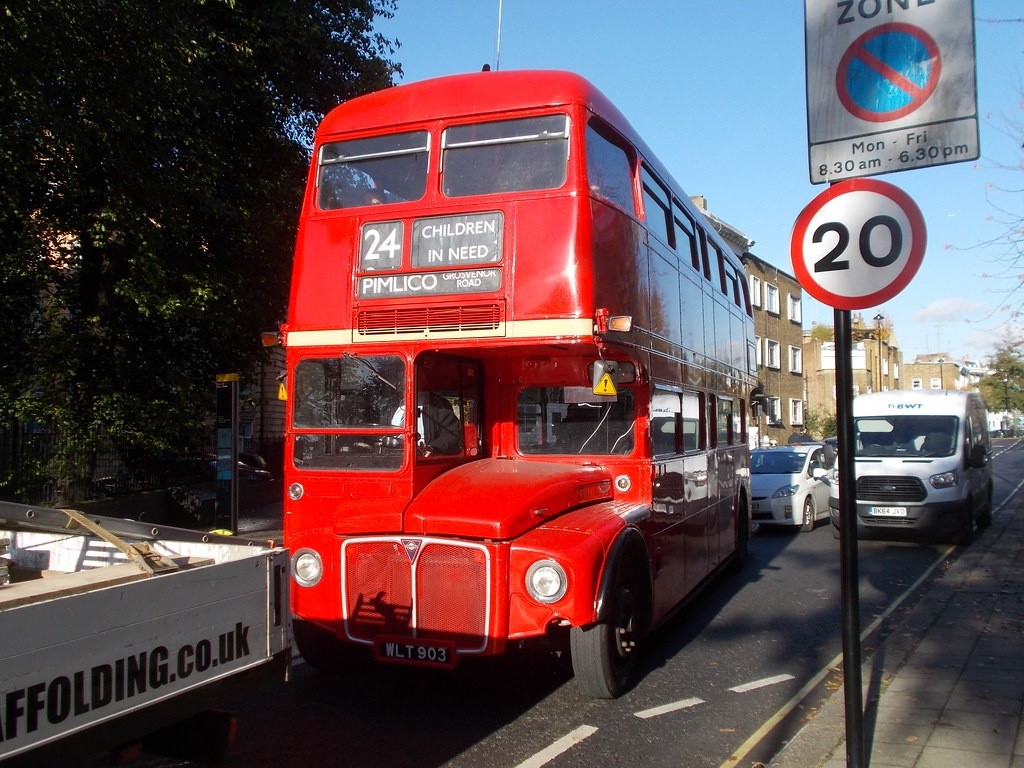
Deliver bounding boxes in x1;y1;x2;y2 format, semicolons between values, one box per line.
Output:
261;67;772;703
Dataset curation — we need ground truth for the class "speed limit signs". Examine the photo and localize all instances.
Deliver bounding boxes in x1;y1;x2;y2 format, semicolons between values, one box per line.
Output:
791;179;929;310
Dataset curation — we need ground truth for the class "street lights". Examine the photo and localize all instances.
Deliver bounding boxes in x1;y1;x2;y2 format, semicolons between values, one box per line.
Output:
214;371;241;540
938;358;946;391
874;314;887;392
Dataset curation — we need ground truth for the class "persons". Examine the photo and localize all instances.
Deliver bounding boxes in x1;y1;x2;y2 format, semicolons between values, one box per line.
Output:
787;429;816;444
382;366;460;455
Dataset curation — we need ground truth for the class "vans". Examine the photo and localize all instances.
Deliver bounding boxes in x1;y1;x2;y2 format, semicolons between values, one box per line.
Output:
826;387;993;543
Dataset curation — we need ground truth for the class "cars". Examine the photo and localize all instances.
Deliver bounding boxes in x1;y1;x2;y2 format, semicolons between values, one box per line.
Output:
742;442;839;533
995;430;1006;439
